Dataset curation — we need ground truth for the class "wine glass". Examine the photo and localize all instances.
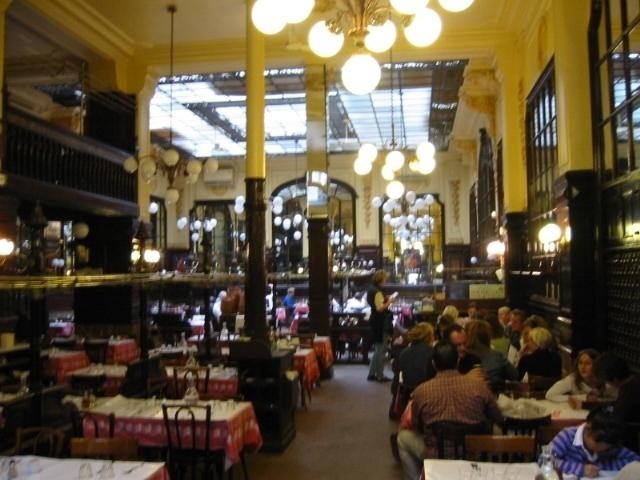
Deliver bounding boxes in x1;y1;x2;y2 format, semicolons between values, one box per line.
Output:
14;371;30;397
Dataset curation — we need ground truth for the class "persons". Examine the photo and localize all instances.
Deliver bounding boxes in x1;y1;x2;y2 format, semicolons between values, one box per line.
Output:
272;296;289;329
218;282;241;332
365;271;394;384
343;291;363;313
383;306;639;479
287;304;310;337
211;284;229;329
327;293;341;315
283;287;297;320
229;339;284;408
234;284;271;334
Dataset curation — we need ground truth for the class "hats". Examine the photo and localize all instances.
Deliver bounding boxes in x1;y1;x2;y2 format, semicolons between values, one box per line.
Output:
407;322;432;340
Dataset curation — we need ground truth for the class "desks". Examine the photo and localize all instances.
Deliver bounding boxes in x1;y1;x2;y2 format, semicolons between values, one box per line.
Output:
421;459;621;480
493;398;588;445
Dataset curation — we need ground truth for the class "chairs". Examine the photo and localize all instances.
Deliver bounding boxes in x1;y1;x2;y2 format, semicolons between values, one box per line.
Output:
425;413;561;462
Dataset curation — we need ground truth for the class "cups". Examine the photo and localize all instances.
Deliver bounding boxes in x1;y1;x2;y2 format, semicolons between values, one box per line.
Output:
1;333;16;350
129;397;236;416
82;390;92;407
160;345;173;353
2;458;116;480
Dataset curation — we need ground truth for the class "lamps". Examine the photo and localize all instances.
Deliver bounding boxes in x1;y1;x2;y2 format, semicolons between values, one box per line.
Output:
124;3;218;208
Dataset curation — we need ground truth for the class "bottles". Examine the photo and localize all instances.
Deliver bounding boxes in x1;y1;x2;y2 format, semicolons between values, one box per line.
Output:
534;446;559;480
179;332;187;352
220;321;228;340
30;239;68;276
109;335;122;345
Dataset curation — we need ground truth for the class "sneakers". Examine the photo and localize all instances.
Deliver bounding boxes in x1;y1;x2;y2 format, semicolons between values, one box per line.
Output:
368;375;376;380
377;376;389;381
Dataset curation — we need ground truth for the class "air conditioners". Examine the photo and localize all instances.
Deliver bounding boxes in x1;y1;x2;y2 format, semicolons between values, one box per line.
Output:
203;168;234;188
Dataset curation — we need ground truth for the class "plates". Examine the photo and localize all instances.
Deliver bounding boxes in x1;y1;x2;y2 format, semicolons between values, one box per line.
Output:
501;407;550;420
